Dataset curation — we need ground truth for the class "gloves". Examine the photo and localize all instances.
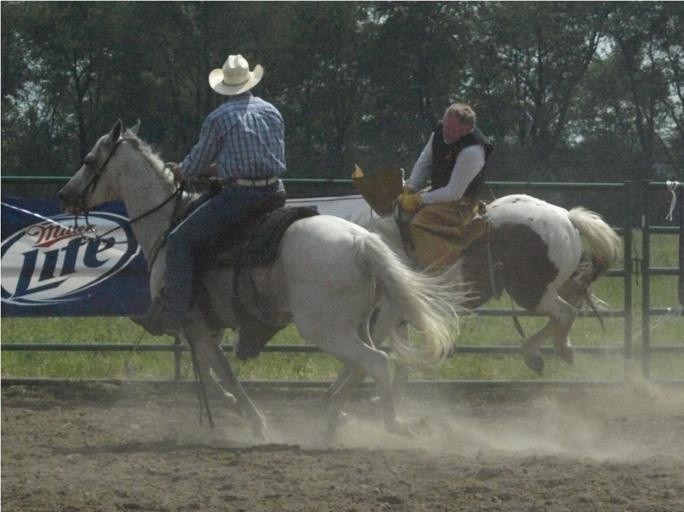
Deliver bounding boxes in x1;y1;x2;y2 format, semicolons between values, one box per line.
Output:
395;187;420;212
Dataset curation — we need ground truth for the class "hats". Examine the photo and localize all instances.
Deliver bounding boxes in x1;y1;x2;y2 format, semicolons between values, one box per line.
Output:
209;54;264;95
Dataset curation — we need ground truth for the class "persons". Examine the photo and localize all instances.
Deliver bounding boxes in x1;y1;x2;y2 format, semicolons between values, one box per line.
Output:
399;103;494;292
130;53;289;335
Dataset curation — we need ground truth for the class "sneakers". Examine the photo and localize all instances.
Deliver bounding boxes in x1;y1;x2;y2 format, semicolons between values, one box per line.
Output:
131;314;164;336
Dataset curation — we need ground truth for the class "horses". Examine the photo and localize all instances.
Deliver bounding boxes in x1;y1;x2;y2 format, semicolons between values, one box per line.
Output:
235;193;625;430
55;118;483;441
